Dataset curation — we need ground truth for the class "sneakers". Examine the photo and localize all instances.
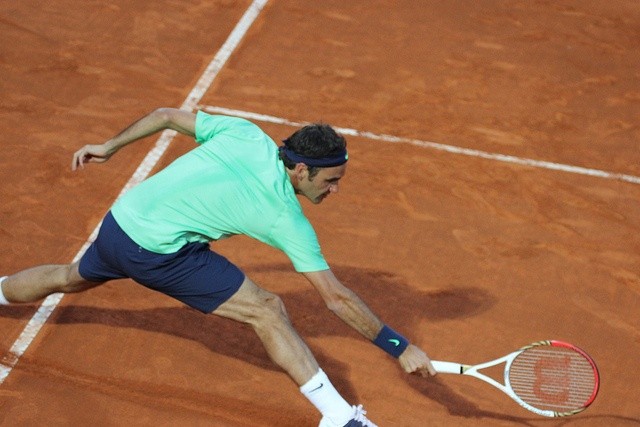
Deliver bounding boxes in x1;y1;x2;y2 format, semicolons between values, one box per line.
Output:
319;404;379;427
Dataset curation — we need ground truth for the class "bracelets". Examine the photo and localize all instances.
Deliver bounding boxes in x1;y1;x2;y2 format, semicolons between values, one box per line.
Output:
372;324;408;358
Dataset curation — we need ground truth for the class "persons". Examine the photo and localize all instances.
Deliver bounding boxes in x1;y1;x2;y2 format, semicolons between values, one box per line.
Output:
0;106;438;427
